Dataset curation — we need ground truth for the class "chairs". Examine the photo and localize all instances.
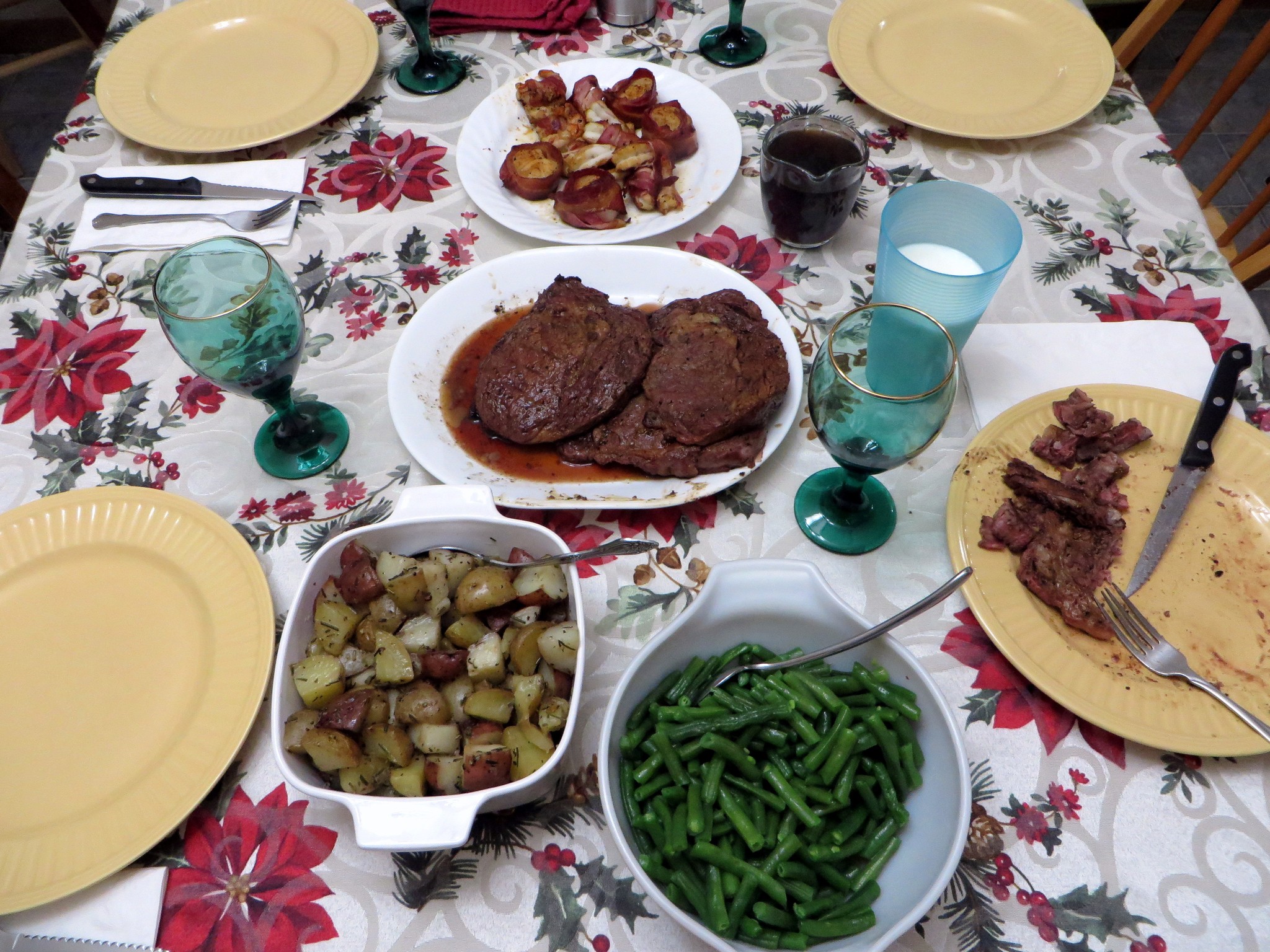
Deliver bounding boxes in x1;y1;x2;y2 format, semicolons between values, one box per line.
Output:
1113;0;1270;297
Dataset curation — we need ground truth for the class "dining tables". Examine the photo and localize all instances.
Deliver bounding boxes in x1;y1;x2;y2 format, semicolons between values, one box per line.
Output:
0;0;1270;950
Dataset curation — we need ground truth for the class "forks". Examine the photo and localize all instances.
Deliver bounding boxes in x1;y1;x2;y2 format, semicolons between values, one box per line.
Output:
1094;581;1270;745
92;195;295;234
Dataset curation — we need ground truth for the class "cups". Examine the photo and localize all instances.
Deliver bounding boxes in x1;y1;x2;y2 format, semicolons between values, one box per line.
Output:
596;0;658;25
762;113;869;252
865;180;1024;402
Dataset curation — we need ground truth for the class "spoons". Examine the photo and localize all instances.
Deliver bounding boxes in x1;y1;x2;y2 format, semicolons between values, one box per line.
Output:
694;565;973;700
407;536;660;569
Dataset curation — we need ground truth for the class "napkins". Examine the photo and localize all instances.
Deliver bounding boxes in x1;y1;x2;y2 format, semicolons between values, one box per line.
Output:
955;317;1250;435
67;158;308;255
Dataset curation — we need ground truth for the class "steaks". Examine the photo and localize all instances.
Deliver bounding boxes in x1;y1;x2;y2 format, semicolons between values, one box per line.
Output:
975;386;1153;638
476;274;790;479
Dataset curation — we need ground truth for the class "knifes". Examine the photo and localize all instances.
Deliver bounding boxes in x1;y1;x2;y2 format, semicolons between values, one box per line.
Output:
80;173;325;202
1121;342;1253;598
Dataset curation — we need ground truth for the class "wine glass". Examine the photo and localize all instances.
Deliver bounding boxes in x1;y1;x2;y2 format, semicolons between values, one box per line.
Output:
699;1;767;68
794;301;957;555
152;237;349;515
385;0;466;94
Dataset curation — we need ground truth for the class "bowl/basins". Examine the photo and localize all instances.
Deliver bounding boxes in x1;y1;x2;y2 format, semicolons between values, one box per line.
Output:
598;559;974;952
272;484;585;849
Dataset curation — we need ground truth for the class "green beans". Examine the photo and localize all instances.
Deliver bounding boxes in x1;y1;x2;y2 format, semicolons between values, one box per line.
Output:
618;640;925;952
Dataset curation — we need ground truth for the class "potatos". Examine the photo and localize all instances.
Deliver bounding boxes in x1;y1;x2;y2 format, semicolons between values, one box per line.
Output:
282;538;582;796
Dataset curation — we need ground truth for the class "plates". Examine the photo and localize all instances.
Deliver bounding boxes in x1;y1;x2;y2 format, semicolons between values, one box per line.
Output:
826;0;1118;141
453;57;747;245
94;0;380;154
0;486;276;920
945;383;1269;757
